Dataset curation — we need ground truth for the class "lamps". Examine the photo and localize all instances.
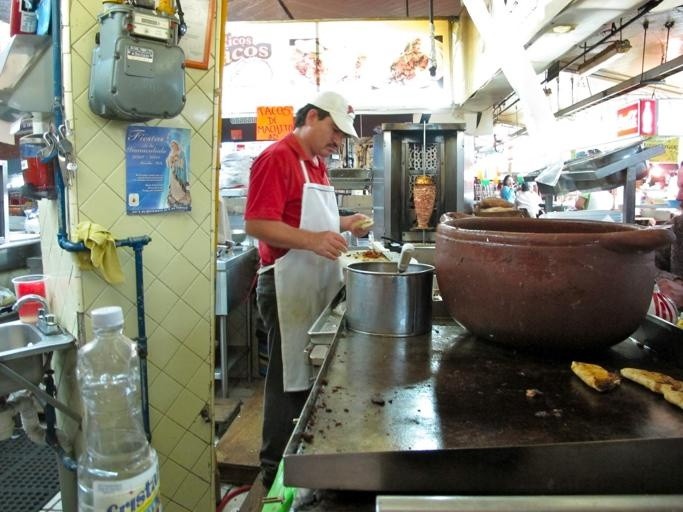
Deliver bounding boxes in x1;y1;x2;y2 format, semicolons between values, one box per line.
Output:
576;18;630;77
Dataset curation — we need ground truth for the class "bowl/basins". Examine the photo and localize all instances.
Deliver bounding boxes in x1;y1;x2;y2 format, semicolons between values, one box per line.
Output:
232;229;246;243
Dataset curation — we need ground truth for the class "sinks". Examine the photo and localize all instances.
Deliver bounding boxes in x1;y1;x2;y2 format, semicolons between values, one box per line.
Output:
0;319;75;397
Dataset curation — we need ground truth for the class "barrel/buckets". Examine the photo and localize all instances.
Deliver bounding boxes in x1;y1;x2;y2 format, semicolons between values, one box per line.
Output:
11;274;49;317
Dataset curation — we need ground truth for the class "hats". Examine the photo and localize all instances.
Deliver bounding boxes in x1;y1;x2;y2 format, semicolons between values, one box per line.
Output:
309;91;360;140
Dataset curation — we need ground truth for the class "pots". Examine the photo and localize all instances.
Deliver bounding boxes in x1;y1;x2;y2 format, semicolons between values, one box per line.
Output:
343;262;435;337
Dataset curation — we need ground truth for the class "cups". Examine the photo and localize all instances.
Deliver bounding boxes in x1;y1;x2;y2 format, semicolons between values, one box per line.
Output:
25;207;40;233
11;274;49;324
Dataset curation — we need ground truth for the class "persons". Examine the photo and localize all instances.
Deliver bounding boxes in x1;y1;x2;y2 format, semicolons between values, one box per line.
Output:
243;92;374;490
575;188;614;210
658;161;683;309
501;177;516;203
166;141;191;208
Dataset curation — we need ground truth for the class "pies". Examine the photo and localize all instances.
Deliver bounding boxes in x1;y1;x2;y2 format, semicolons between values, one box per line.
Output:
621;368;683;409
572;360;620;392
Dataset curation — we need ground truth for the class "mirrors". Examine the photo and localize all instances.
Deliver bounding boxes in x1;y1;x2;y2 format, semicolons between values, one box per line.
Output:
1;0;56;323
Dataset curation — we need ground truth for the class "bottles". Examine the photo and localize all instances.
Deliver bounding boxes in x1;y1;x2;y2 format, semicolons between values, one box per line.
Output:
75;305;162;511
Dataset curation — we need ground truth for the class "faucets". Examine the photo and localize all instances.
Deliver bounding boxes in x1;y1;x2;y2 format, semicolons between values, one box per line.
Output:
12;294;59;335
